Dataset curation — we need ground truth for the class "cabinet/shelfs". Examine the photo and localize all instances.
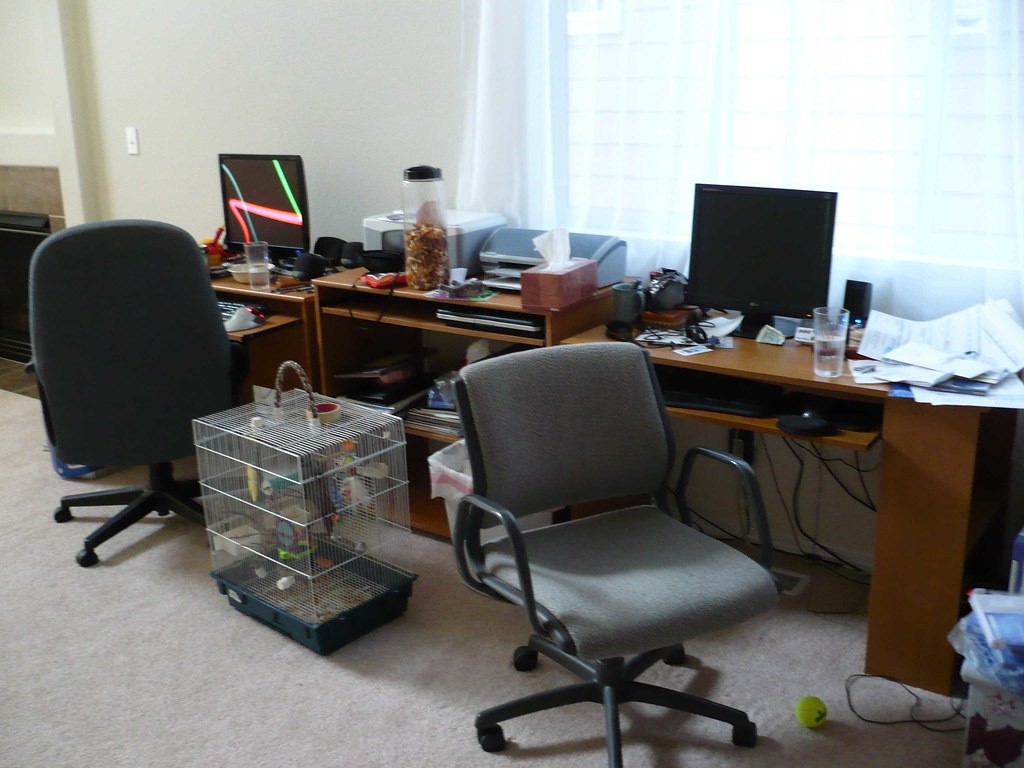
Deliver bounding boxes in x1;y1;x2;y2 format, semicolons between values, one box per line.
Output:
311;267;641;538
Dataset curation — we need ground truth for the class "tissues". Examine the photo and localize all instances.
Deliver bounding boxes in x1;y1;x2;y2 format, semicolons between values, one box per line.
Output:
520;227;599;313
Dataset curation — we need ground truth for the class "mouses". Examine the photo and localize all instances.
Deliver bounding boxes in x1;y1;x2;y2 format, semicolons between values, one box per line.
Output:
686;324;708;344
242;304;270;324
803;409;826;420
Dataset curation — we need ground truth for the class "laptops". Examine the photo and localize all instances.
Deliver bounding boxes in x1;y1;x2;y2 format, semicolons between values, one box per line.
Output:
436;307;545;338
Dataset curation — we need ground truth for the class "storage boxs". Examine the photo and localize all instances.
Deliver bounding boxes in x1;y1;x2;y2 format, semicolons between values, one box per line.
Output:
361;208;506;276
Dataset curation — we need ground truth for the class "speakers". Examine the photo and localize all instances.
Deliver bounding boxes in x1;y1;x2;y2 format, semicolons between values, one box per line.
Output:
842;280;872;327
292;236;366;281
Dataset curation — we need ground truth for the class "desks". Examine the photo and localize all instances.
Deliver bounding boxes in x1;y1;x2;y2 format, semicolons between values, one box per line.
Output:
558;326;1024;698
210;276;317;393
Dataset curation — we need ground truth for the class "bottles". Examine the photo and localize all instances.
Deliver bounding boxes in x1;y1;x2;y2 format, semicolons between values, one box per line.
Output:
402;164;451;290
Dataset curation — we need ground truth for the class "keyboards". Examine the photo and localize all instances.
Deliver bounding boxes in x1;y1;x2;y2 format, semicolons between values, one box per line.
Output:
216;299;247;322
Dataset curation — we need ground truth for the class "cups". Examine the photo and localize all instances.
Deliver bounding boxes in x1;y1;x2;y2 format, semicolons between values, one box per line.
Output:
612;283;646;323
244;241;270;290
812;307;850;378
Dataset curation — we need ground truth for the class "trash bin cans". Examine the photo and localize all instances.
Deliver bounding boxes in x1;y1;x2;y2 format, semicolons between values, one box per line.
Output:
426;437;554;545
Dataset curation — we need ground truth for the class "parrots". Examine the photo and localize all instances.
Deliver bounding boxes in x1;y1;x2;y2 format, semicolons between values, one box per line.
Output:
247;464;371;534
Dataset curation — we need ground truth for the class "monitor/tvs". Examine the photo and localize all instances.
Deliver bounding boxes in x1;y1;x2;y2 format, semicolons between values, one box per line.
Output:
219;153;311;272
686;184;838;340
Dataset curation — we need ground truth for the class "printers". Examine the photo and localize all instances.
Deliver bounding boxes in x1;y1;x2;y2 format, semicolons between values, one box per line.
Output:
477;227;628;291
363;207;508;280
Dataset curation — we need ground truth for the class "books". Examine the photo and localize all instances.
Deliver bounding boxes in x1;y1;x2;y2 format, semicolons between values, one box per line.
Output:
329;345;466;441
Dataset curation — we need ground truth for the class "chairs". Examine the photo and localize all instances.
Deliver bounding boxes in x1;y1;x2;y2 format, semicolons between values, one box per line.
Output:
450;340;757;768
28;218;251;568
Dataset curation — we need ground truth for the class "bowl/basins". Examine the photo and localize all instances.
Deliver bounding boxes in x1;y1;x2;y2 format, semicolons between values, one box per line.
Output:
227;263;275;283
